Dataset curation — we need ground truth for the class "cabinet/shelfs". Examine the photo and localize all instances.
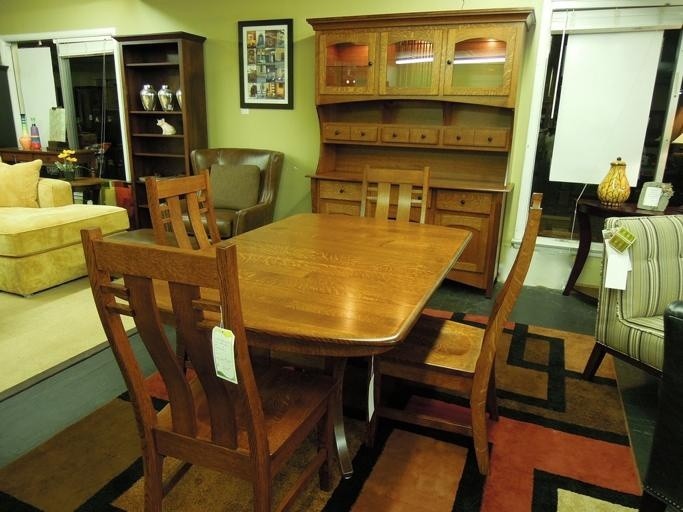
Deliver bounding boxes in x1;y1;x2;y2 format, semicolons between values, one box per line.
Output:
303;7;536;298
114;30;207;227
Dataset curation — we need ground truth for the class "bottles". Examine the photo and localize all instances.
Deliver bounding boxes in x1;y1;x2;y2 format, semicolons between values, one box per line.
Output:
18;113;31;150
30;117;41;150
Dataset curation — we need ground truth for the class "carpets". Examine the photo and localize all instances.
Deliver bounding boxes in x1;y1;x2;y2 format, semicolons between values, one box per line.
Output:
1;308;643;512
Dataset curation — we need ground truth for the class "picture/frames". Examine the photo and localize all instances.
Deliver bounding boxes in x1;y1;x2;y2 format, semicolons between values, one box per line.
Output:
237;20;295;112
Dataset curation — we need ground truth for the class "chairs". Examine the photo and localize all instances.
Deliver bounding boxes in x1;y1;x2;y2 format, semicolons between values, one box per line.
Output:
145;169;221;373
81;225;341;512
369;192;543;474
360;164;431;228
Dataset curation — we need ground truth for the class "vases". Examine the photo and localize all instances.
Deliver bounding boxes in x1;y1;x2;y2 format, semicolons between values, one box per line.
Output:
62;172;76;181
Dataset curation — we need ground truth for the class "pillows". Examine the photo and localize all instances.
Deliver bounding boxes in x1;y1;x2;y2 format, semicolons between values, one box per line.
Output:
209;163;261;211
0;159;42;209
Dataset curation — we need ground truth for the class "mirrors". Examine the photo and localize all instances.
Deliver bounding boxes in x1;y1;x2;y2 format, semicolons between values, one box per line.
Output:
11;41;63;148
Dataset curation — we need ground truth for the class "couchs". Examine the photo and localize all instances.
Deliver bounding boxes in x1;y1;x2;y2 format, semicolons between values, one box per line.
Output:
159;147;284;236
0;178;130;301
582;214;683;378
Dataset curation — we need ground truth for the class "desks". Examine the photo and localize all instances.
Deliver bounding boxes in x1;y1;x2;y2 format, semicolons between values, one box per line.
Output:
106;212;473;485
562;198;683;303
1;148;95;176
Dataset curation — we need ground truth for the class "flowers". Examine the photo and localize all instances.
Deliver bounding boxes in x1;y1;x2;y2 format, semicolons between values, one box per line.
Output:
54;149;78;171
